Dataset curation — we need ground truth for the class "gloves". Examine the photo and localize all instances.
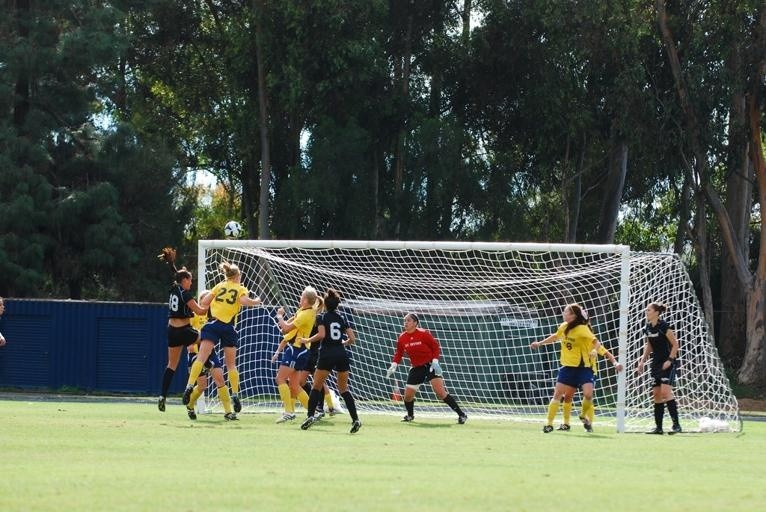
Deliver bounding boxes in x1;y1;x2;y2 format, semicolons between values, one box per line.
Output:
386;364;397;378
430;359;441;375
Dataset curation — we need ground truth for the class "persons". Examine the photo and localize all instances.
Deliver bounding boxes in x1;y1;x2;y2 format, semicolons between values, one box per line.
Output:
1;295;8;346
556;324;624;433
158;247;362;434
528;303;601;433
634;302;681;435
384;312;468;425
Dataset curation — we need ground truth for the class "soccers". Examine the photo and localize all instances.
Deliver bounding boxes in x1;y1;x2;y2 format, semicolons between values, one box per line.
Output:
224;220;243;239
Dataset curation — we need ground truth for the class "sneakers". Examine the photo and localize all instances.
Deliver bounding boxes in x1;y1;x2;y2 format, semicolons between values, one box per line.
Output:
668;426;681;435
459;414;467;424
350;421;362;433
158;396;166;411
646;429;663;434
276;408;337;430
400;416;414;422
224;395;241;421
543;414;593;434
182;387;197;421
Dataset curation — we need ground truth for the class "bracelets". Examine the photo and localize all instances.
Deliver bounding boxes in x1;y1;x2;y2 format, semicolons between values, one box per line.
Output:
612;361;621;367
667;356;675;363
